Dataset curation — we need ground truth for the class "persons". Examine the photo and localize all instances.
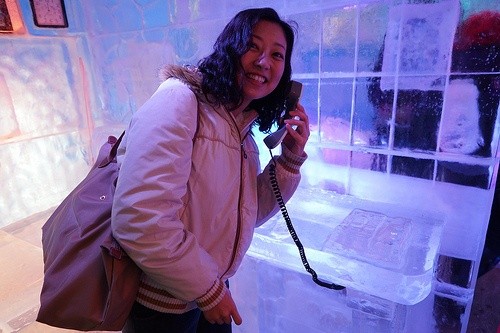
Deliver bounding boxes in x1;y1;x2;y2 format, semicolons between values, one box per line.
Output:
109;7;310;333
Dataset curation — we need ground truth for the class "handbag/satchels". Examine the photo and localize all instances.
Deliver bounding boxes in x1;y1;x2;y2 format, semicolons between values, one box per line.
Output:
34;134;143;332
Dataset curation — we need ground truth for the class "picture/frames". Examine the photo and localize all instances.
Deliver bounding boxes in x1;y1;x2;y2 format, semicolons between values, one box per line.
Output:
0;0;14;33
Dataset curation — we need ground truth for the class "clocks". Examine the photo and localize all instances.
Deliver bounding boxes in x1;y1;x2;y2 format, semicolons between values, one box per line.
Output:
29;0;68;28
